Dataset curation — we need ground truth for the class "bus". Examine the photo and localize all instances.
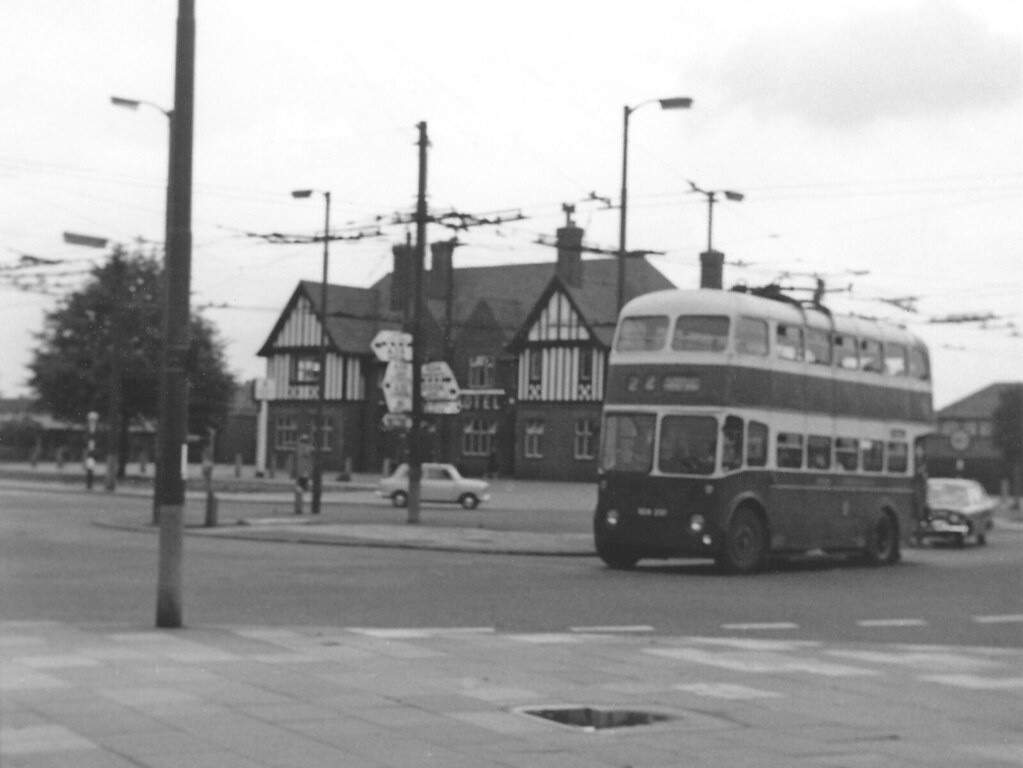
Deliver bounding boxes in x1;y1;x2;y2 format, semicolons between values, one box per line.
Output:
592;284;934;575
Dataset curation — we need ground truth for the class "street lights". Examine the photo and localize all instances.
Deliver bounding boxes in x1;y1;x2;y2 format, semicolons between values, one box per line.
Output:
612;93;693;342
60;229;143;495
292;184;333;514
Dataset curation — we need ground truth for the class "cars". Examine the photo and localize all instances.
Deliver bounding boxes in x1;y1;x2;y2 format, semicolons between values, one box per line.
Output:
375;459;490;509
919;475;995;547
